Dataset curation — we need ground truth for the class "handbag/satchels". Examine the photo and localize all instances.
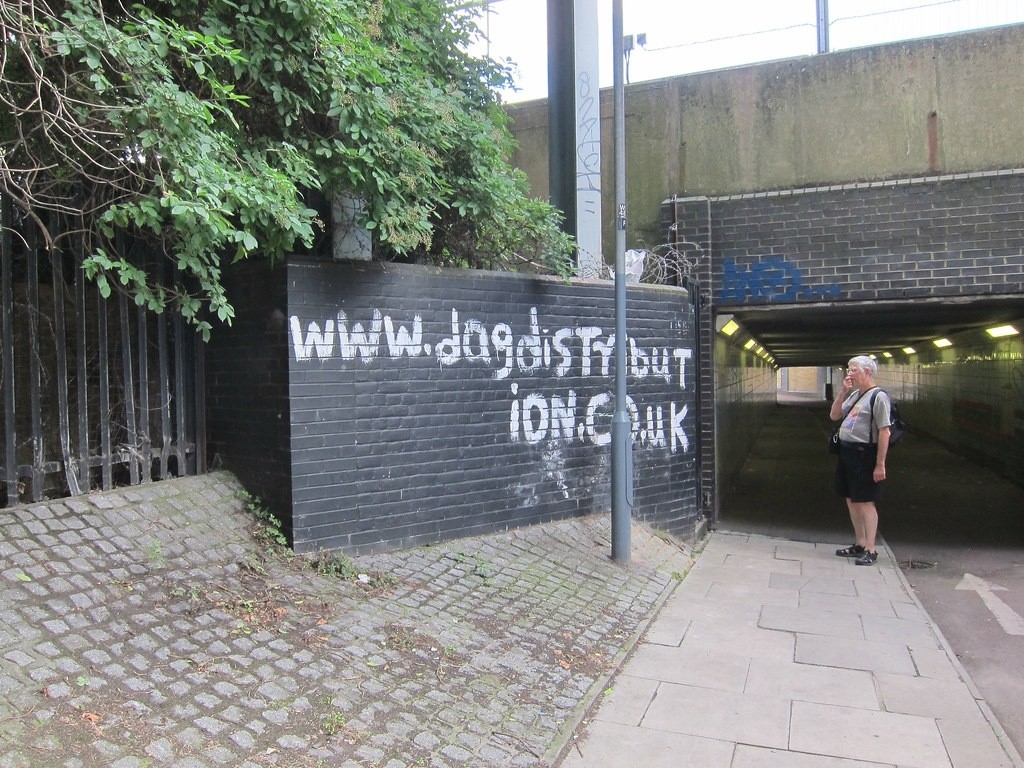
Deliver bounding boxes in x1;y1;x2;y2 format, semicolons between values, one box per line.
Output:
829;388;906;453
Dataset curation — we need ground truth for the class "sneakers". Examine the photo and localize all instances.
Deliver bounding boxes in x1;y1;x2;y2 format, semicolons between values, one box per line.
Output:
856;550;878;565
836;543;865;556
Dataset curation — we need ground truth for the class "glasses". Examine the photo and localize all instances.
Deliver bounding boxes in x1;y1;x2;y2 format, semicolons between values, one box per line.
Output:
845;368;860;374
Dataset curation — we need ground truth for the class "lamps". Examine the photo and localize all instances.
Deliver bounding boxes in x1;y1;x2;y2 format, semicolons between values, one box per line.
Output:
716;314;779;371
869;318;1024;360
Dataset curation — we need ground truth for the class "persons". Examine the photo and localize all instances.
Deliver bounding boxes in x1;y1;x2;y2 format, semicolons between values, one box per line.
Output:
828;356;891;566
